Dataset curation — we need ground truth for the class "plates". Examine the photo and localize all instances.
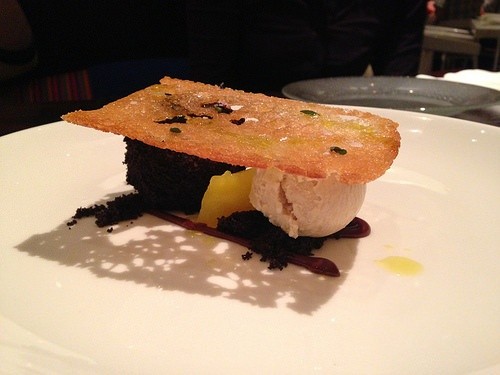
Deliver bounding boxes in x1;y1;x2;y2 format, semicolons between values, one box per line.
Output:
2;103;500;375
282;76;500;117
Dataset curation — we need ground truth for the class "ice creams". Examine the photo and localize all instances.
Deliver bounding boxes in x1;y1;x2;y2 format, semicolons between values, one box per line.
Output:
67;136;365;273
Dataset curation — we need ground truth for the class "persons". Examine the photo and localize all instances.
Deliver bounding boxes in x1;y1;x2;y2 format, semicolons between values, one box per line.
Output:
244;0;439;78
0;0;38;85
